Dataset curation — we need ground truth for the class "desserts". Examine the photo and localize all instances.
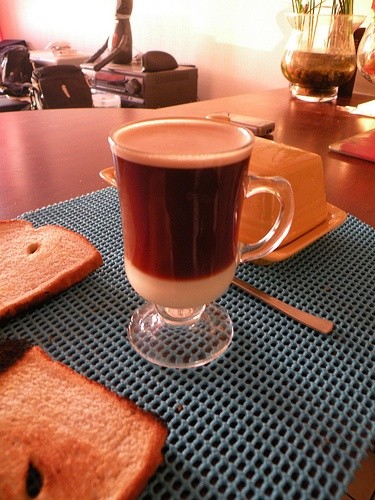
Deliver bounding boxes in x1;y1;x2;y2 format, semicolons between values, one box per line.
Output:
240;138;329;253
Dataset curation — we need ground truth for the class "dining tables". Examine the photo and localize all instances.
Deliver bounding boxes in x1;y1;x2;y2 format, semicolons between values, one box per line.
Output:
0;87;375;500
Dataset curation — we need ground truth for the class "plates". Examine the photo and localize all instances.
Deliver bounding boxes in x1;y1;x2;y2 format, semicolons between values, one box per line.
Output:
98;167;347;262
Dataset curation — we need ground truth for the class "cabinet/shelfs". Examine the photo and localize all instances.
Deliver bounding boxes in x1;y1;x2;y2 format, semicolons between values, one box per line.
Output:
79;63;199;110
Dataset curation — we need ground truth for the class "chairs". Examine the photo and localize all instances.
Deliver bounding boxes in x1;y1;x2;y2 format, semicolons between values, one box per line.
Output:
27;65;96;111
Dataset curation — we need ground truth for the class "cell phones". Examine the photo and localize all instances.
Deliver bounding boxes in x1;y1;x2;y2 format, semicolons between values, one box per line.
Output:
205;113;275;135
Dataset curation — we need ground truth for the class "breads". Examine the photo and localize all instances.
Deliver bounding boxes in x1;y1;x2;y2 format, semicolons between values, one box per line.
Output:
0;341;172;500
0;219;102;323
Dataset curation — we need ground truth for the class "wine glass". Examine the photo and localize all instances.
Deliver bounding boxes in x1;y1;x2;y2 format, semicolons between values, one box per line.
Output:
108;118;294;369
